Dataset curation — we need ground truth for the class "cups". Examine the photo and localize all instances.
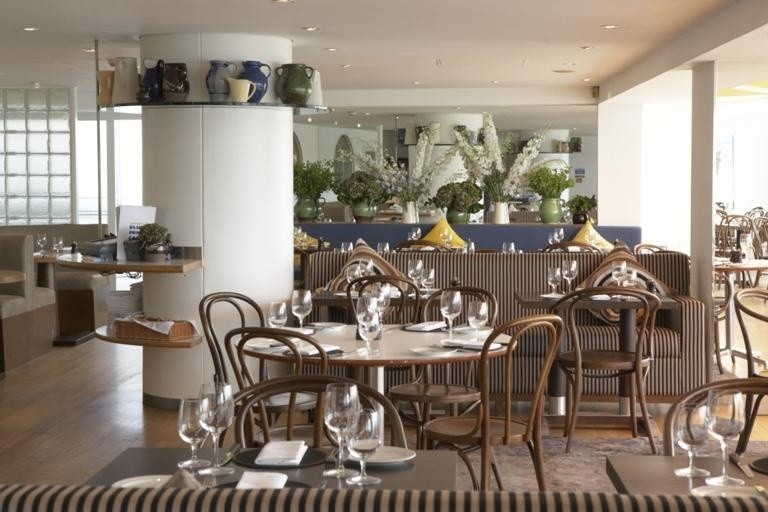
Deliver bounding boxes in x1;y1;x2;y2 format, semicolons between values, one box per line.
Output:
268;302;287;330
626;267;636;287
225;76;256;103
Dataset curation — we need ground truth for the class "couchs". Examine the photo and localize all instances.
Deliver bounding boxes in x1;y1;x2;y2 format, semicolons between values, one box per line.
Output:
0;483;768;512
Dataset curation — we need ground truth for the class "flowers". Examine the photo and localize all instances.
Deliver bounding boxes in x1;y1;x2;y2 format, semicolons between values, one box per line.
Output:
337;126;458;201
451;113;552;203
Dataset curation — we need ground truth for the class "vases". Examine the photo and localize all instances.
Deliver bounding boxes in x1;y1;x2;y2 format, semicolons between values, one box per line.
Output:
97;56;314;107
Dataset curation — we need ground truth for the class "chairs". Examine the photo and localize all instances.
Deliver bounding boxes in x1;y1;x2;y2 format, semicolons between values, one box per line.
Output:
715;200;768;259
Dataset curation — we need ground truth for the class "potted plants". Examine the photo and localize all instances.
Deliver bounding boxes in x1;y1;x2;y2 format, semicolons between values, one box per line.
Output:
565;194;597;224
294;159;336;220
140;223;168;261
333;171;390;220
423;180;486;224
529;168;575;224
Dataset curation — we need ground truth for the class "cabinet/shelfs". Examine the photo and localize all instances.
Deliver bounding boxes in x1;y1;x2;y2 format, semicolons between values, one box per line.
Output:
56;254;202;348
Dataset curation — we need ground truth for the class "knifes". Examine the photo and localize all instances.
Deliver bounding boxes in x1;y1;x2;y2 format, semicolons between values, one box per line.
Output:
729;453;754;479
219;444;241;466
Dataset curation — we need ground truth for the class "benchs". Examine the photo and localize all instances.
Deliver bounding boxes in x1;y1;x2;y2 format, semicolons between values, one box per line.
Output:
0;224;117;377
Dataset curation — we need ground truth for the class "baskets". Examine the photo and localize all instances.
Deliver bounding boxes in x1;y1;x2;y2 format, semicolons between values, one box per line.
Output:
115;315;193;341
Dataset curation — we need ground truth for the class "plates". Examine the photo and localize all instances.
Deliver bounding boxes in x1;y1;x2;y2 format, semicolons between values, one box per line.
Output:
111;474;172;489
541;293;566;299
691;486;759;497
442;340;499;352
347;446;417;466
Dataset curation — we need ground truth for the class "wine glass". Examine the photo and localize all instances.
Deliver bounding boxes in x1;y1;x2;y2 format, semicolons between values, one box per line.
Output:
290;289;312;328
52;237;63;259
674;403;711;478
422;267;436;296
740;233;752;264
611;260;625;287
563;260;577;292
467;301;488;340
343;258;393;351
546;266;562;294
440;290;463;341
37;234;48;256
176;399;211;469
408;259;423;296
704;390;745;487
198;383;235;475
345;407;381;486
322;383;359;477
